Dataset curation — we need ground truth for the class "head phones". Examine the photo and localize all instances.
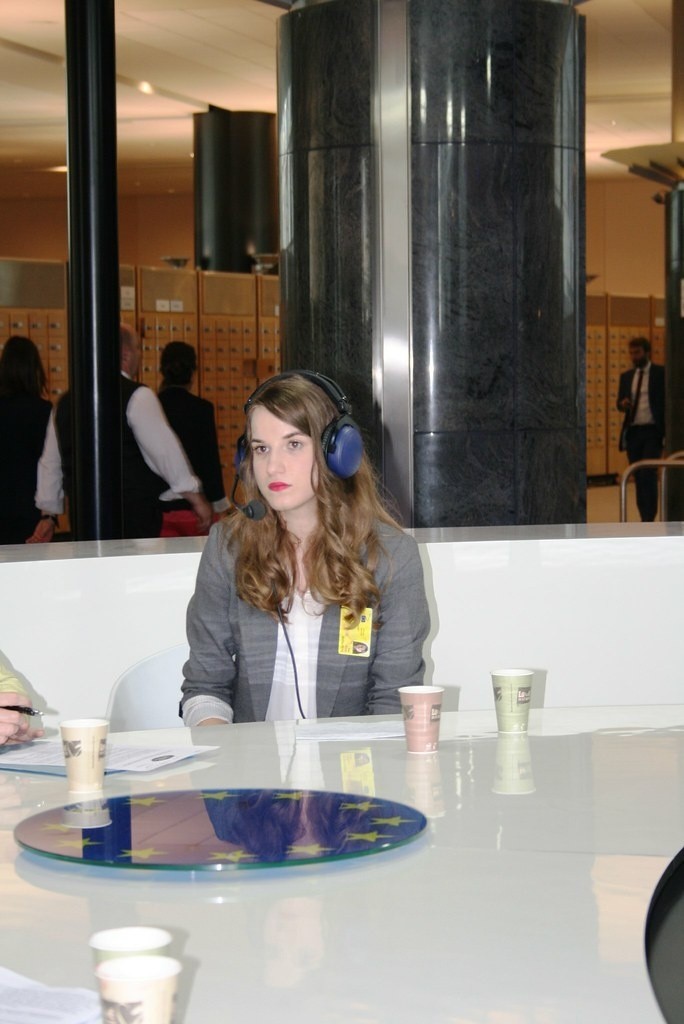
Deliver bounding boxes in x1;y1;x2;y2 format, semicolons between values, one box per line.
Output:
234;370;364;481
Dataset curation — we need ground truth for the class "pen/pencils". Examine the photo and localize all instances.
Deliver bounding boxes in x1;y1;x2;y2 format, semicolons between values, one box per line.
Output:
0;704;47;717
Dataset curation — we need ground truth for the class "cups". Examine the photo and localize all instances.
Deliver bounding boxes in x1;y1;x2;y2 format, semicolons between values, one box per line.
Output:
60;718;109;792
398;686;444;753
492;667;534;734
87;925;183;1024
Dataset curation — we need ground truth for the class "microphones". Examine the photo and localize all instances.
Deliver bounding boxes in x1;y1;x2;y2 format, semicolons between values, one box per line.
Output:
230;474;267;523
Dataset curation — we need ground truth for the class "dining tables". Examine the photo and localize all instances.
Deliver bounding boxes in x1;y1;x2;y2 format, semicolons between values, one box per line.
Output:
0;700;683;1023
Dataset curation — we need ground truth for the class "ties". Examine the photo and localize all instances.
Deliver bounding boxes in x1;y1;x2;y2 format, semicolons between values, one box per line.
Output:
628;369;644;424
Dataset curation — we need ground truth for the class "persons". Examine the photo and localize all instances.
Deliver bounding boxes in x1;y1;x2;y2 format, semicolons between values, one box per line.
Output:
619;338;665;527
24;326;211;548
176;371;434;739
0;661;46;751
0;334;60;541
154;342;228;544
